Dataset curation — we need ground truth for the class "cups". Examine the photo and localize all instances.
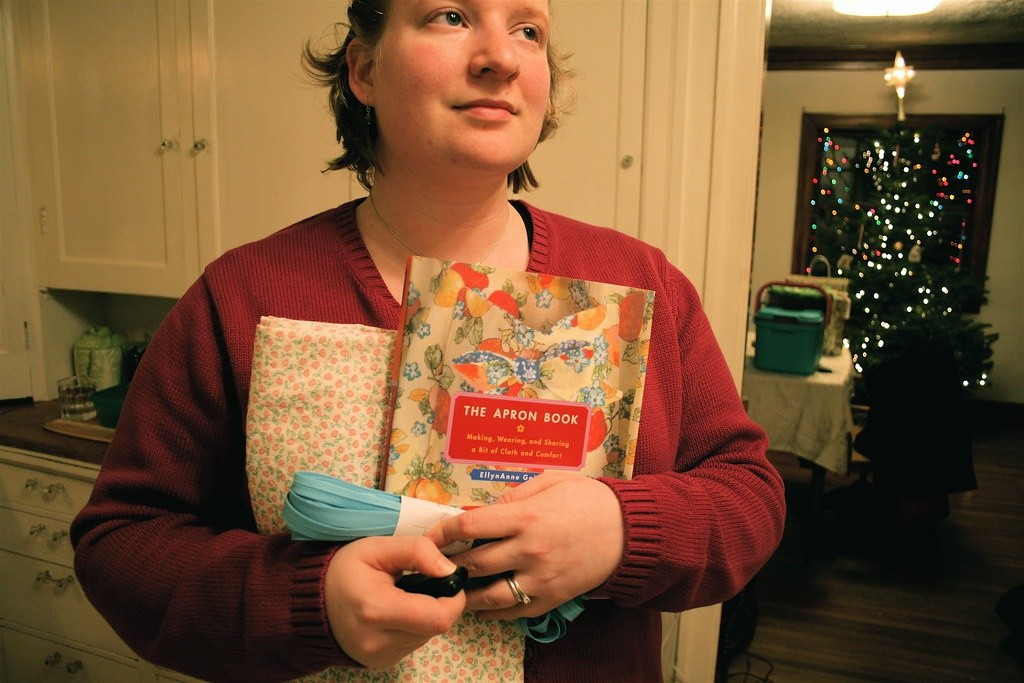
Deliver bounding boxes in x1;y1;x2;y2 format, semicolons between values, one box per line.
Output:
58;377;98;420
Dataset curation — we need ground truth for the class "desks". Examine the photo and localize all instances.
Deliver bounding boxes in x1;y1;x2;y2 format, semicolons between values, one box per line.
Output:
739;330;854;567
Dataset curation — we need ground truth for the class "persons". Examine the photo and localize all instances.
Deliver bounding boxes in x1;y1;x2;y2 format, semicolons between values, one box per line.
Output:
68;0;787;683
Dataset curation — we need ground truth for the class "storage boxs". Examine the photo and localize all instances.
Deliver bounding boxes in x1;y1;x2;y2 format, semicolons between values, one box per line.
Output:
753;280;832;374
785;273;849;298
823;294;852;356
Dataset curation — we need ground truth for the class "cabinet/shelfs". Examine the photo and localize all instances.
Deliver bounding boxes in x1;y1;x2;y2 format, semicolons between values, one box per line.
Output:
0;446;208;682
507;0;650;240
0;26;31;401
22;0;349;298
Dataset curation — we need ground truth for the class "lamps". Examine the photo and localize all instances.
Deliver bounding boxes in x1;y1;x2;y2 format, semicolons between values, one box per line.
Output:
883;50;914;122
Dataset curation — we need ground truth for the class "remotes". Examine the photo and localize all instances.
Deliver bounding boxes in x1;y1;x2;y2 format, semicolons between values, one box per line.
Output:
394;566;468;599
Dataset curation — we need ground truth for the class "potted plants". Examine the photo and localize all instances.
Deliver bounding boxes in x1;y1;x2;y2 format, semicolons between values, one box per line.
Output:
856;307;1001;522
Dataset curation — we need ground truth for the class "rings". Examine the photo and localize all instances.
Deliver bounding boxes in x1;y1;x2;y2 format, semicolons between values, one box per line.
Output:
506;576;532;604
